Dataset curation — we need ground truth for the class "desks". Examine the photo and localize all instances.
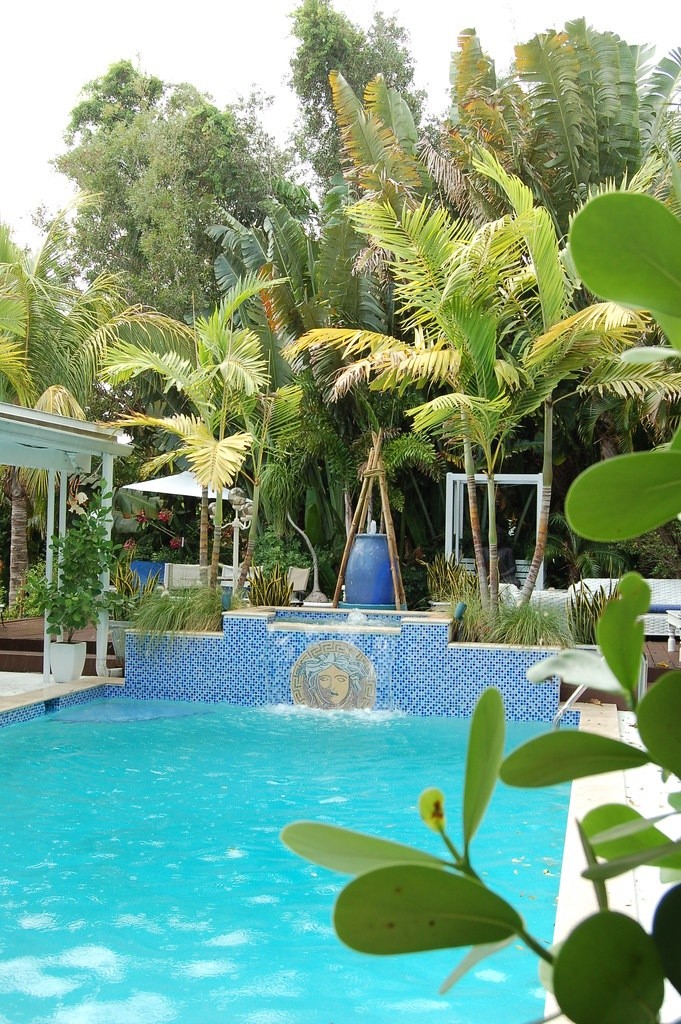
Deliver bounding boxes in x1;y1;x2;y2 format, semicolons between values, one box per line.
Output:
666;609;681;662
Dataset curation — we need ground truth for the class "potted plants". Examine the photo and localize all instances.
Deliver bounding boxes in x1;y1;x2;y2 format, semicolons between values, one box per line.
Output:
414;551;469;611
25;477;123;683
109;547;167;667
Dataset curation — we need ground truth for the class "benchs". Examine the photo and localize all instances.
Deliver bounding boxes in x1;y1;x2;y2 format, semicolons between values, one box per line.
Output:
459;558;536;592
568;578;681;642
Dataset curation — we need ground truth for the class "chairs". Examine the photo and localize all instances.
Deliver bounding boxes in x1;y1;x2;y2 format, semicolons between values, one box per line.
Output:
498;583;568;624
